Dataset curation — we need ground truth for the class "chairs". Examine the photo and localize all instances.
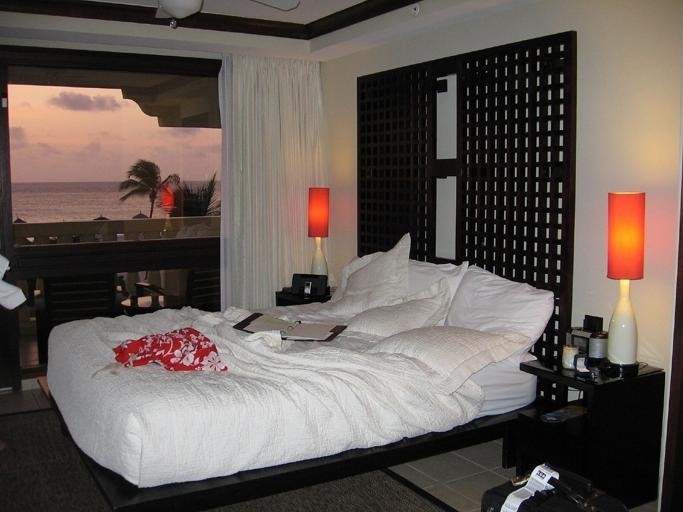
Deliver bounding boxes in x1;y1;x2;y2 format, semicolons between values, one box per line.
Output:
34;273;129;365
134;267;221;312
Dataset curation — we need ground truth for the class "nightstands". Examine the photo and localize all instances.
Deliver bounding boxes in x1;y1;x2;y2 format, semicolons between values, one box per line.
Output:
275;288;335;307
517;355;666;508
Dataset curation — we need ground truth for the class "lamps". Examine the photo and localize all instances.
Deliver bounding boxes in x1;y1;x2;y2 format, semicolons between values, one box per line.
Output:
608;193;644;377
307;188;330;285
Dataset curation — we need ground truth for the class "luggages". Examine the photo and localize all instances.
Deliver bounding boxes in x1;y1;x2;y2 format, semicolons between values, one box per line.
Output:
480;462;628;512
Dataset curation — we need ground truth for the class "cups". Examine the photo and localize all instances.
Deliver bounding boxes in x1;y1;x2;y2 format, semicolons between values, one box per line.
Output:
130;295;138;307
93;232;104;243
72;232;81;244
25;237;34;245
562;346;579;368
116;233;125;241
152;295;159;306
159;225;170;238
48;236;58;244
136;232;144;241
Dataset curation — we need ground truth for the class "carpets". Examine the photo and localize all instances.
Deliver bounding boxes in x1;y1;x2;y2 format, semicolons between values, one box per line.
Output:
0;406;456;512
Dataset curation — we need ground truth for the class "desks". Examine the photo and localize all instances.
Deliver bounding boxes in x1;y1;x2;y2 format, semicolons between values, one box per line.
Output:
121;292;188;317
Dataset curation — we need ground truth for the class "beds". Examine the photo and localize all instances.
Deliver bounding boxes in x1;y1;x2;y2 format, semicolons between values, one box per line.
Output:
48;252;572;512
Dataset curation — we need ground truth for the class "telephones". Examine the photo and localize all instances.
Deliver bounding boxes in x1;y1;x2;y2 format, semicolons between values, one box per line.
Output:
303;281;312;300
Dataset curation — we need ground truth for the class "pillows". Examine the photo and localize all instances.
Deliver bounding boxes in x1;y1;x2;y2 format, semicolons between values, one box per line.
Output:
345;277;450;340
330;258;468;326
365;325;531;395
317;232;411;318
444;265;555;368
